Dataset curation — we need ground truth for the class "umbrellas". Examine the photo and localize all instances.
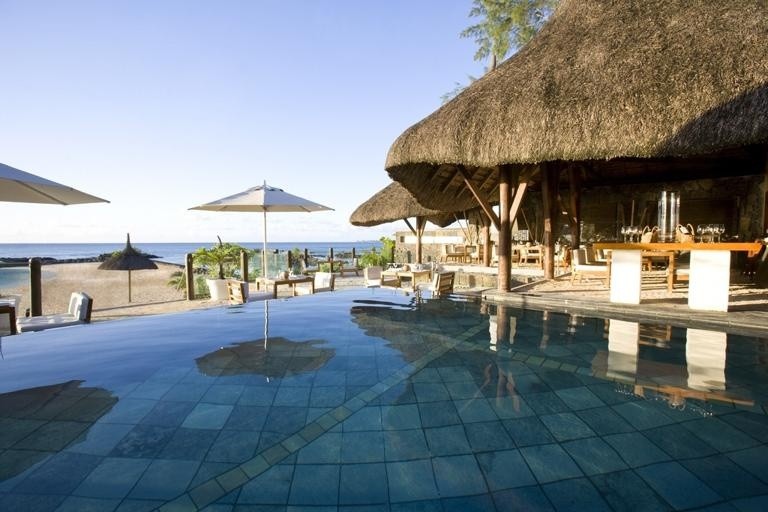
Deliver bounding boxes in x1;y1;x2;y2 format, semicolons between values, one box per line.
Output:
1;379;119;484
193;297;336;388
0;163;112;206
184;178;336;293
97;234;159;302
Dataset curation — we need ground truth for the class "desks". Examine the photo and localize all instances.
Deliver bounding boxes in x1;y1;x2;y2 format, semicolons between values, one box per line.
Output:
593;242;762;312
1;296;16;335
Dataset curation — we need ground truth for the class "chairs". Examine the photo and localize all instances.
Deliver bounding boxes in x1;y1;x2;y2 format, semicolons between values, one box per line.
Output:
16;291;93;333
570;242;611;288
364;265;455;294
440;241;569;266
227;272;335;305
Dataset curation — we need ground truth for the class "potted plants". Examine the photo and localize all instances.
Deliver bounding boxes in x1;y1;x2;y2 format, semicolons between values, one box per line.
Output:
192;236;254;301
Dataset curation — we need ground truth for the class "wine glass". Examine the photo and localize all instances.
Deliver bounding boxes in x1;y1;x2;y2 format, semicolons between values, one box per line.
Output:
620;223;726;244
